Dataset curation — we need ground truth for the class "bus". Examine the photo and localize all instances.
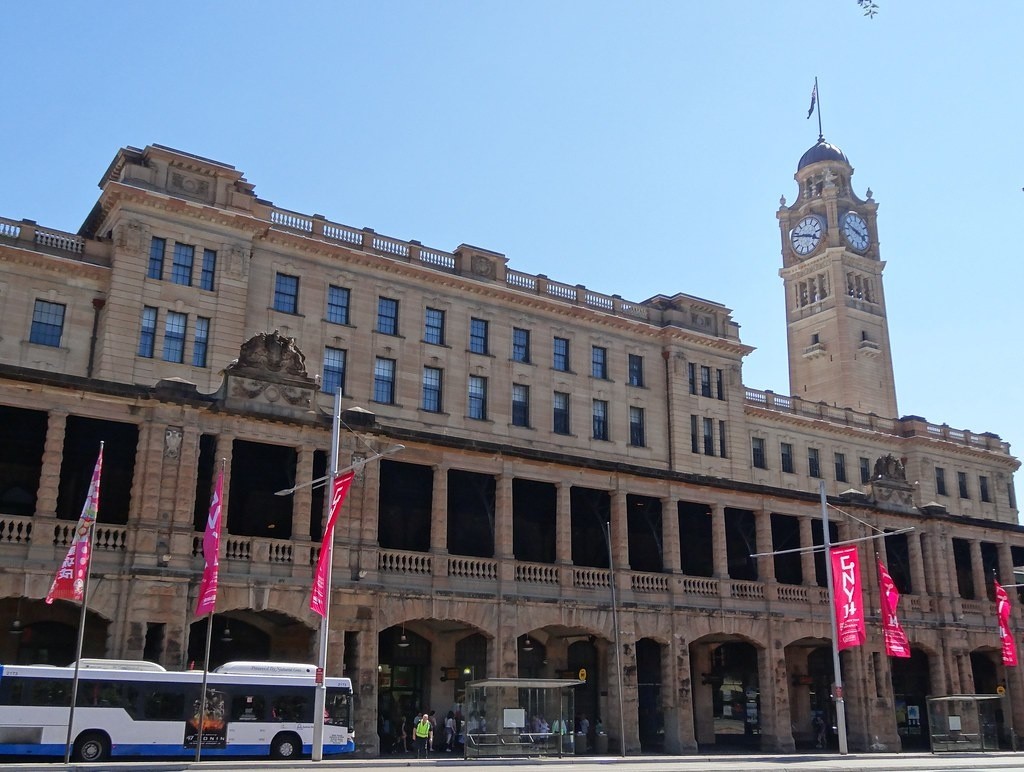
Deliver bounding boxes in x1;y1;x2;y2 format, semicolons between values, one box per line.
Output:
0;657;356;764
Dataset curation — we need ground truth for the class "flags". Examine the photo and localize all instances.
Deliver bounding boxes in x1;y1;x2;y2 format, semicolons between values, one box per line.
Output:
807;85;816;118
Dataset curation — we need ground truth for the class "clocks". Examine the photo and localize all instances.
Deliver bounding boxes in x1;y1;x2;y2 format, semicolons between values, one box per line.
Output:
842;209;872;254
789;214;828;260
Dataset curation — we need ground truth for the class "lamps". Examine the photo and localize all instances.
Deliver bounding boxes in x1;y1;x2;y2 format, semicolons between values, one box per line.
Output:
221;613;234;644
398;624;410;648
9;600;25;635
522;632;533;652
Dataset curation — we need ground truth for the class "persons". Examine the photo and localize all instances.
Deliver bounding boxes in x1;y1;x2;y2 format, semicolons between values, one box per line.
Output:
390;711;604;758
